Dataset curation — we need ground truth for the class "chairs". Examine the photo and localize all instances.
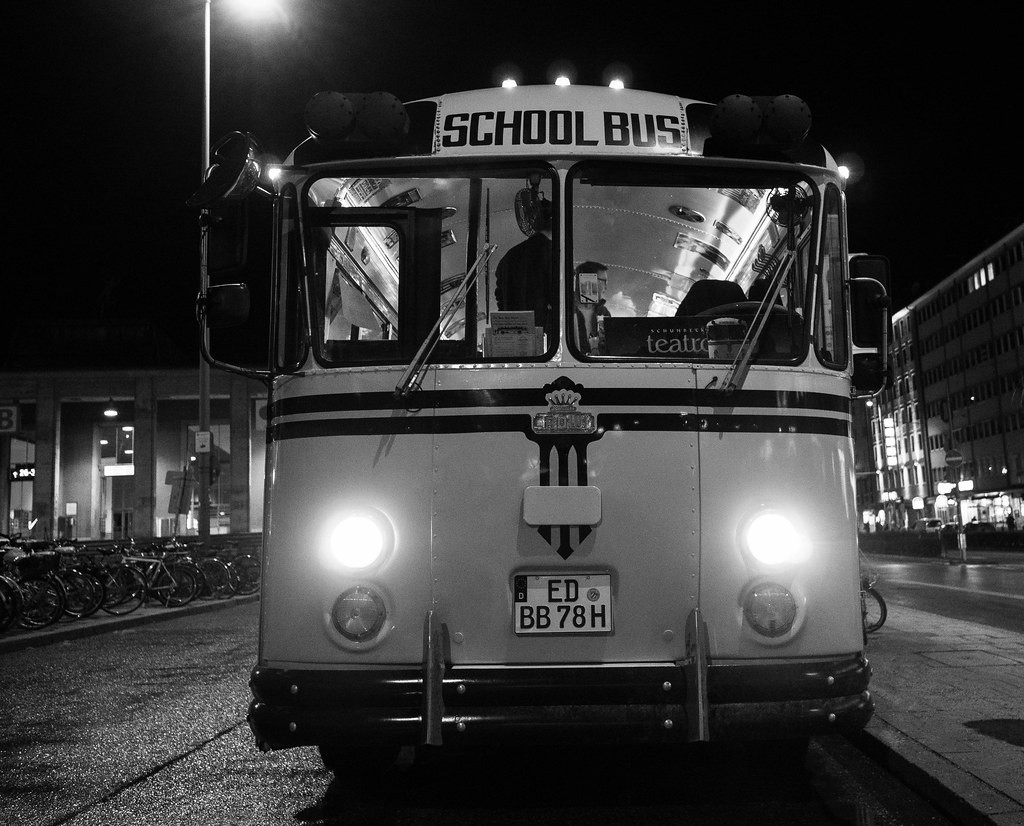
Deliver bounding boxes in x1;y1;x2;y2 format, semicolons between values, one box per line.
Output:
674;279;748;316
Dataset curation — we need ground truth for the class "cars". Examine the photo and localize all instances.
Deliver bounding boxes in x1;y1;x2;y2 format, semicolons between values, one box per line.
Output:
964;522;995;534
940;523;959;534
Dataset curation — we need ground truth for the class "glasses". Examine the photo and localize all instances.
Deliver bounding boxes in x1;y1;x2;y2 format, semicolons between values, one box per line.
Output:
598;277;608;285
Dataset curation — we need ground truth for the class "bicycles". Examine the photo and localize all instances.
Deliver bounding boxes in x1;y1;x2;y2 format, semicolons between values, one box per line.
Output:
0;534;264;633
858;547;886;647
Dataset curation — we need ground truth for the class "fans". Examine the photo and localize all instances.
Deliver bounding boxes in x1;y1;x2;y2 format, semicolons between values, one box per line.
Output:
515;174;544;238
765;183;809;250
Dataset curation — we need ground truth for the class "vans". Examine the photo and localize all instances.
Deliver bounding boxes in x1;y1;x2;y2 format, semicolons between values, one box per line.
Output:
909;518;942;533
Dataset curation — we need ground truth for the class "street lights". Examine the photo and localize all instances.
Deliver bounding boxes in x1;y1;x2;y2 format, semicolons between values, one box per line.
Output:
866;391;888;530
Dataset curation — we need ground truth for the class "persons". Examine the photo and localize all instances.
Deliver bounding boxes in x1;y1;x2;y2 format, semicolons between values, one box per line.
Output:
1006;514;1016;531
495;200;553;343
576;262;612;355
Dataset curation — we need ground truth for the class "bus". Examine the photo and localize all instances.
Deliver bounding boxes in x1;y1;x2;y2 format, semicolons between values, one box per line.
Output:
194;82;890;785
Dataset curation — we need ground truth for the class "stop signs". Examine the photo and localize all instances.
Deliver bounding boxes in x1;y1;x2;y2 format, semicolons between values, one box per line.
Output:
944;450;964;468
